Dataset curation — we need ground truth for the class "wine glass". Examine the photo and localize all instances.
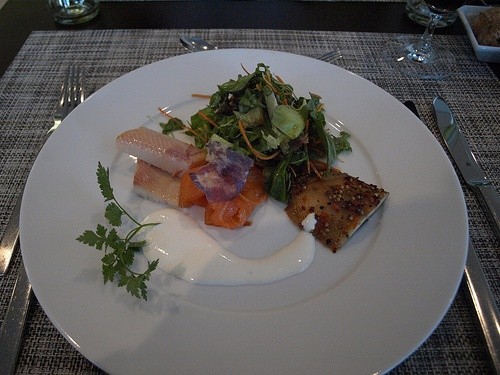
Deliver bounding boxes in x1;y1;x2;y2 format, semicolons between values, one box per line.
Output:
382;0;456;80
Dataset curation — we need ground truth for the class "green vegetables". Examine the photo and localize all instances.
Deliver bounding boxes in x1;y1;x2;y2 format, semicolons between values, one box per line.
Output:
76;161;162;300
155;60;353;202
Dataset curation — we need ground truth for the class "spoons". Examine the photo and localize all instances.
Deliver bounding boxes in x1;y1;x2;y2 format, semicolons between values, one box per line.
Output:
180;35;218;53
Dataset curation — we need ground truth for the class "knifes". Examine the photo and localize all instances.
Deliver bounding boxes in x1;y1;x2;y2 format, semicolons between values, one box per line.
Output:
432;95;500;231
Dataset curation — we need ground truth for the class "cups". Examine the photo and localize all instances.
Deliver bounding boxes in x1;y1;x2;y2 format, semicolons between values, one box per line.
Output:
47;0;95;25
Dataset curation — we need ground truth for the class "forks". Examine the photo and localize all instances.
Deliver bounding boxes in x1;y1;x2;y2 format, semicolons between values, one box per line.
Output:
316;49;343;64
0;64;85;375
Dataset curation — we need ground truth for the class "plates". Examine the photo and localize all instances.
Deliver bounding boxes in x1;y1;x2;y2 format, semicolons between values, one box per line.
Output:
457;5;500;61
20;49;468;374
